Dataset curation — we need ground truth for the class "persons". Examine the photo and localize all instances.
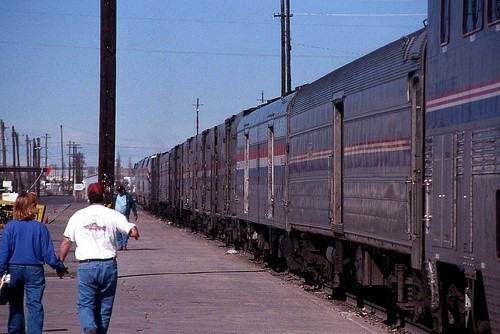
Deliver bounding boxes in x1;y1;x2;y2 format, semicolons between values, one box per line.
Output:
0;192;69;334
104;186;139;251
57;182;140;334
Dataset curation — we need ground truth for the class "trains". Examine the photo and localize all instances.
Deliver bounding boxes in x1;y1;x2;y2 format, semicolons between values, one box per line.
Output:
129;0;500;334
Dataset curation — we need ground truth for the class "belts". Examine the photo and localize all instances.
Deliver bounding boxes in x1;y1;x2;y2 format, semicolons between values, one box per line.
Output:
79;257;115;263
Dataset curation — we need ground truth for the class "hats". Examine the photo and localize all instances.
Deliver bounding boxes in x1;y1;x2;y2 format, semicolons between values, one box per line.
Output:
87;182;105;197
116;185;123;191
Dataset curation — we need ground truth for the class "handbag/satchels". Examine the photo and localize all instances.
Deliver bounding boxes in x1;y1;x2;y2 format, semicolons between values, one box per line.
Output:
0;266;11;305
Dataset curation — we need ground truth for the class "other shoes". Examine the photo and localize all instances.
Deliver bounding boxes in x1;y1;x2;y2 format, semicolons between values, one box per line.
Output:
118;246;123;250
123;247;128;250
88;328;97;334
